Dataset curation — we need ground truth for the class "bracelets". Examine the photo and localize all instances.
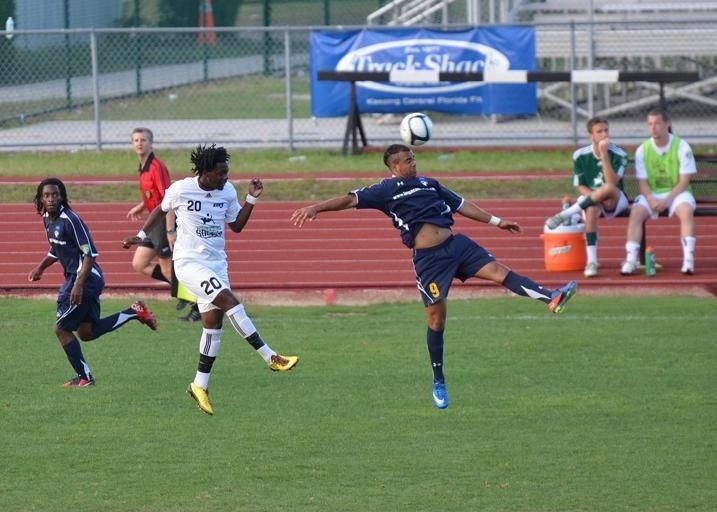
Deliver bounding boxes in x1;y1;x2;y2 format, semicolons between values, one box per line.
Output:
486;214;502;228
246;191;261;207
135;228;148;241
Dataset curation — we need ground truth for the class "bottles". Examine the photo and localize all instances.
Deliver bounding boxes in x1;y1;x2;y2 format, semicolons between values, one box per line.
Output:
645;245;656;277
560;195;572;225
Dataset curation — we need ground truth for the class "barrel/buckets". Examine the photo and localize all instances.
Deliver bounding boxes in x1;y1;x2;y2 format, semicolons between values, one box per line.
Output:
543;223;585;272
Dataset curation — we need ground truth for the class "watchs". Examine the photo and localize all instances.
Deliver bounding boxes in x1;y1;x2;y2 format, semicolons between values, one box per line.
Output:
165;228;175;234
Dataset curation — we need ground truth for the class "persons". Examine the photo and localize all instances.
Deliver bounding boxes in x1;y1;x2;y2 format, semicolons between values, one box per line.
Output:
27;177;159;389
619;109;699;277
121;143;299;416
286;144;577;408
125;125;200;322
543;116;629;274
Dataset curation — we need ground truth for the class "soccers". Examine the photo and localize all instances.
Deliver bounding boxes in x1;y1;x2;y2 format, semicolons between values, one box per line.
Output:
399;113;433;145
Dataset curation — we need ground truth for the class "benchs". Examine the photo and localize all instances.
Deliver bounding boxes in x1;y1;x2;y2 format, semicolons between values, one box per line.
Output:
580;158;716;270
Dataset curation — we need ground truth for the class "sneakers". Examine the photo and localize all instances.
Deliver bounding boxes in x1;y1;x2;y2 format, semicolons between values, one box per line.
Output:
59;376;96;389
176;298;201;322
268;354;299;371
430;381;449;410
548;279;576;313
547;212;565;230
584;261;599;277
186;382;214;417
131;299;158;333
621;261;637;274
681;260;696;274
